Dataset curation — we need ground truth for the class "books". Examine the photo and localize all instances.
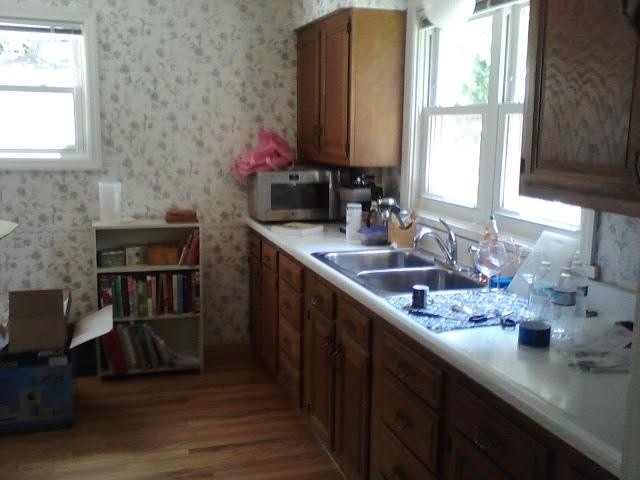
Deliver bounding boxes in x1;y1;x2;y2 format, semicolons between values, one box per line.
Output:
98;232;200;375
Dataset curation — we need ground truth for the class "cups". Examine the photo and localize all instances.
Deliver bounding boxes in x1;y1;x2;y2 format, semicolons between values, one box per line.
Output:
368;197;396;229
410;285;433;312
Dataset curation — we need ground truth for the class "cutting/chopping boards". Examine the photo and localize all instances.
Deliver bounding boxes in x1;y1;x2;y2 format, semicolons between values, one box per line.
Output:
505;228;578;316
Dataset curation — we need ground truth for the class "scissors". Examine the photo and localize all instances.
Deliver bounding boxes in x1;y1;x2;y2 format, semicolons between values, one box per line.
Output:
468;307;517;328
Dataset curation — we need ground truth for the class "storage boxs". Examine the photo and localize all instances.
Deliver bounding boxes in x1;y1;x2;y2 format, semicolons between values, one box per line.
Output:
0;302;113;438
3;288;73;352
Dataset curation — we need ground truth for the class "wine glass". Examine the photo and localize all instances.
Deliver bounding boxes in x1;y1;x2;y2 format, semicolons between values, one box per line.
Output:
474;236;531;304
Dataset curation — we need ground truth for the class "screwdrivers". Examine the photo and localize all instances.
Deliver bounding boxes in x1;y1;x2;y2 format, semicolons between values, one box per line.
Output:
408;310;467;322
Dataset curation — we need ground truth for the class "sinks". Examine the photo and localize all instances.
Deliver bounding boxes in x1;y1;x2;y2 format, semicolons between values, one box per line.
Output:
322;250;436;273
357;267;486;296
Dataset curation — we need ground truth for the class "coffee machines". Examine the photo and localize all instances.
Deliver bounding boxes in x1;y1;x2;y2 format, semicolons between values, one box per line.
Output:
339;171;391;238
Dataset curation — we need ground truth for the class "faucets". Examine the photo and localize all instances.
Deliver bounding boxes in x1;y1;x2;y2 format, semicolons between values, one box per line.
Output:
412;216;458;265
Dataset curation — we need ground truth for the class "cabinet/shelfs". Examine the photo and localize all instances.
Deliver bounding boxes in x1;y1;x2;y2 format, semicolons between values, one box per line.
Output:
445;380;580;478
90;217;209;387
306;277;374;474
294;7;406;169
247;228;301;409
513;1;639;216
367;332;443;478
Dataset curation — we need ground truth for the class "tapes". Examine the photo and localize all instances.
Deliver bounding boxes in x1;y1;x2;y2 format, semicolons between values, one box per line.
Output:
519;322;550;348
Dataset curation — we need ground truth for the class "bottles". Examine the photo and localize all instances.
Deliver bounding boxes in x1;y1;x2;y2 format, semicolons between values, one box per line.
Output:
346;202;364;239
531;260;588;340
484;215;497;259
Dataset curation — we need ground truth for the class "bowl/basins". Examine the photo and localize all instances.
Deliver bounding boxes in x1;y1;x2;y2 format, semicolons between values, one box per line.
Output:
357;229;387;245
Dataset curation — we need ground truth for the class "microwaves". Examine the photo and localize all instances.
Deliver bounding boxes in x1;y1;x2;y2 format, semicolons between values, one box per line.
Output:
249;165;352;222
